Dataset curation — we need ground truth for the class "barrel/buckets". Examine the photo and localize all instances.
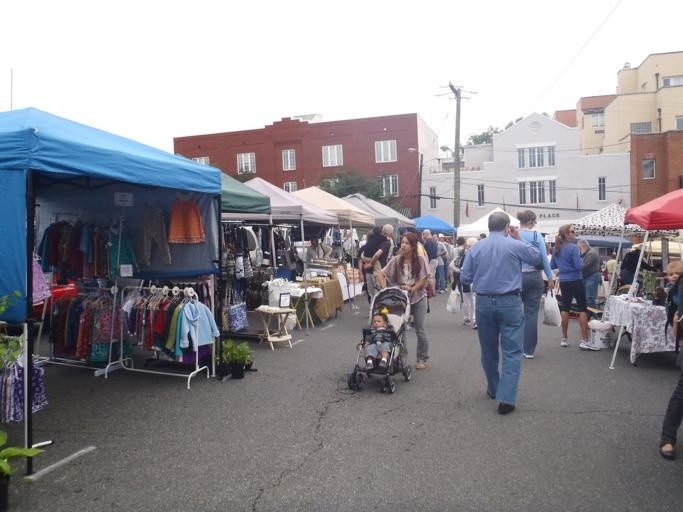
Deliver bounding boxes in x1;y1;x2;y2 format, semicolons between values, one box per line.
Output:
586;319;613;350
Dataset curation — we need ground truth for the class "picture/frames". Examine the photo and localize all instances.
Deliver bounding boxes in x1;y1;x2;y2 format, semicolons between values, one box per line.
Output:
277;292;291;308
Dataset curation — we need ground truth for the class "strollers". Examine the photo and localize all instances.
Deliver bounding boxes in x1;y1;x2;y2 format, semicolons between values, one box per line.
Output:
346;286;411;392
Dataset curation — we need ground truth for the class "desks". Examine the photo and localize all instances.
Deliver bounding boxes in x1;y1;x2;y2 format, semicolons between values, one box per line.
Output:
600;291;680;371
306;262;366;303
291;285;322;330
300;275;344;325
255;303;299;353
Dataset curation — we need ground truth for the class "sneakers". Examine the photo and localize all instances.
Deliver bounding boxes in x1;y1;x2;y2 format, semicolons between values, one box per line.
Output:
365;363;373;370
473;323;477;329
560;338;569;347
579;342;600;351
499;403;514;414
416;362;428;369
378;361;387;368
464;318;471;326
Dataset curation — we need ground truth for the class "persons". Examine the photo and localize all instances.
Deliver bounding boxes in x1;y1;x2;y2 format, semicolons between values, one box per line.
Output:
460;211;543;414
378;232;432;370
506;211;555;359
272;255;293;280
362;312;397;369
541;243;663;297
550;224;600;351
306;209;486;330
659;271;682;460
576;238;602;321
665;261;682;348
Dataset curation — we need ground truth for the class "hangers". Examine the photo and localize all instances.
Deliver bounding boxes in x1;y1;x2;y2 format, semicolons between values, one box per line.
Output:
46;211;121;233
48;283;213;313
0;335;50;369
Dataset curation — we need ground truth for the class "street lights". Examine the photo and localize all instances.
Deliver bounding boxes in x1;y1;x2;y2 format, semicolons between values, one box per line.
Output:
440;145;459;227
407;147;423;216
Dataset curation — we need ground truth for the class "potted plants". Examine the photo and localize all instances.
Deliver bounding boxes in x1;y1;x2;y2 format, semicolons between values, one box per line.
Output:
0;429;47;512
219;336;254;382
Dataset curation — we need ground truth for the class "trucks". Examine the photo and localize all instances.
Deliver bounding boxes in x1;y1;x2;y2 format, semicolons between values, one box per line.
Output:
275;222;331;260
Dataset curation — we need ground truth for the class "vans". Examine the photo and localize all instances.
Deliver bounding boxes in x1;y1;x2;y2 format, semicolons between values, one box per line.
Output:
220;225;288;276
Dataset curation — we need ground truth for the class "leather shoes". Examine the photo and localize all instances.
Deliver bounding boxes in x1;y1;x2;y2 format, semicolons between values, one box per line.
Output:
659;440;675;460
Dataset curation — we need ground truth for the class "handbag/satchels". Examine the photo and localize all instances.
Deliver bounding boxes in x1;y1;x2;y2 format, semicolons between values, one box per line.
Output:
221;302;249;333
277;249;299;271
221;249;255;281
532;241;544;270
427;281;434;298
447;285;460;313
